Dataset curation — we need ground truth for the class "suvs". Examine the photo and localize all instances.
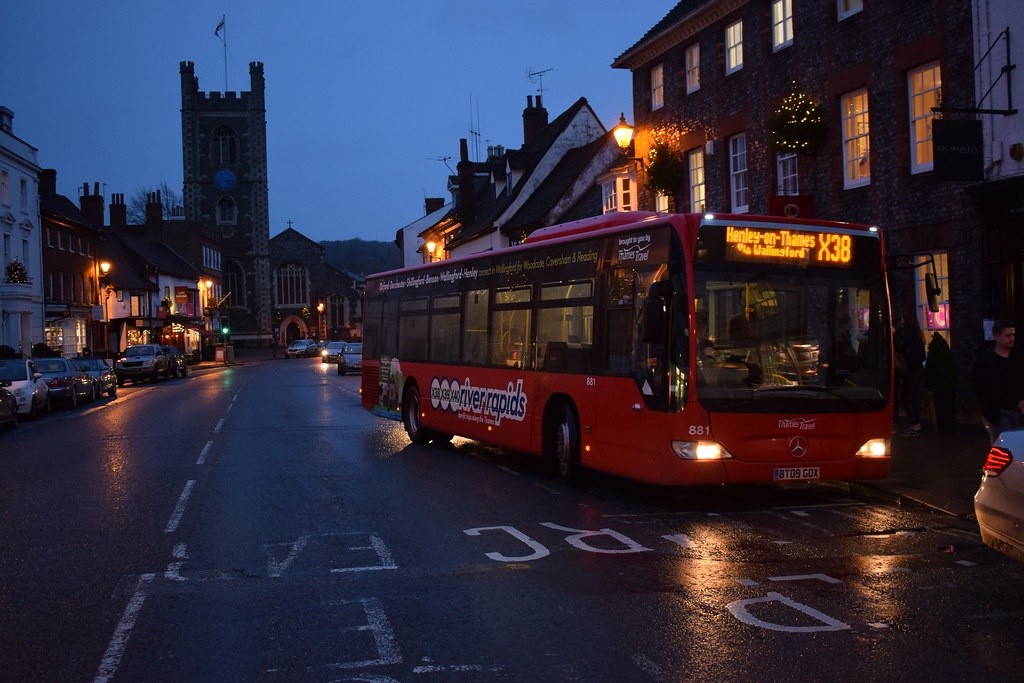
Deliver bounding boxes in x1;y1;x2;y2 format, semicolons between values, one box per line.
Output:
115;343;169;386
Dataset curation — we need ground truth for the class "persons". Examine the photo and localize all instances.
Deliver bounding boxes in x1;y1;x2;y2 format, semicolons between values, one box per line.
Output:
671;313;715;369
287;334;293;345
894;312;926;437
976;319;1024;444
827;305;872;375
728;315;755;349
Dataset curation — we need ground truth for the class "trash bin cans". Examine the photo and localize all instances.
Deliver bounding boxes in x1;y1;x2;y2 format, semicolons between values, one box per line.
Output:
226;346;234;361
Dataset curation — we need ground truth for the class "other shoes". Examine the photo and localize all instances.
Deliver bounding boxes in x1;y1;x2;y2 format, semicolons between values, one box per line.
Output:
898;428;921;437
905;420;928;433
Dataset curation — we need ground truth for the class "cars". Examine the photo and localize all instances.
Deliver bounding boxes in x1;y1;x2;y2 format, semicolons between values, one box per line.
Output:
285;339;318;358
314;340;331;354
972;428;1023;563
32;358;95;408
1;357;53;418
160;347;189;377
336;343;363;376
71;358;116;399
321;342;347;363
0;380;19;429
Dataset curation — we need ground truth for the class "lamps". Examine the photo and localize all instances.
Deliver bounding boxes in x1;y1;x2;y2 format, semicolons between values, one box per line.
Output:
426;237;441;259
611;112;644;167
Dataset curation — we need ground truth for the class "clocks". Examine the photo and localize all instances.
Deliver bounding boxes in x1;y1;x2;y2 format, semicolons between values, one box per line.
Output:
214;169;238;193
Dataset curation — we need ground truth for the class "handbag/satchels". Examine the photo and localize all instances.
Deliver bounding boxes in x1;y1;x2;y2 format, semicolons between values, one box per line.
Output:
894;351;907;371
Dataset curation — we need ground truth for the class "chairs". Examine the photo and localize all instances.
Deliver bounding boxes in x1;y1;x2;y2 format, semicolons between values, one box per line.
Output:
49;365;61;370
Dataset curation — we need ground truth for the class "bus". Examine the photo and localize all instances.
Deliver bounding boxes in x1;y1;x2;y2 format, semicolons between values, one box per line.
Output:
362;208;943;492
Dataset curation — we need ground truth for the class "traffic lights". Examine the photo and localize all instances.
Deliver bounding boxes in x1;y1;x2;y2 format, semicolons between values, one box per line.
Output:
221;316;229;335
